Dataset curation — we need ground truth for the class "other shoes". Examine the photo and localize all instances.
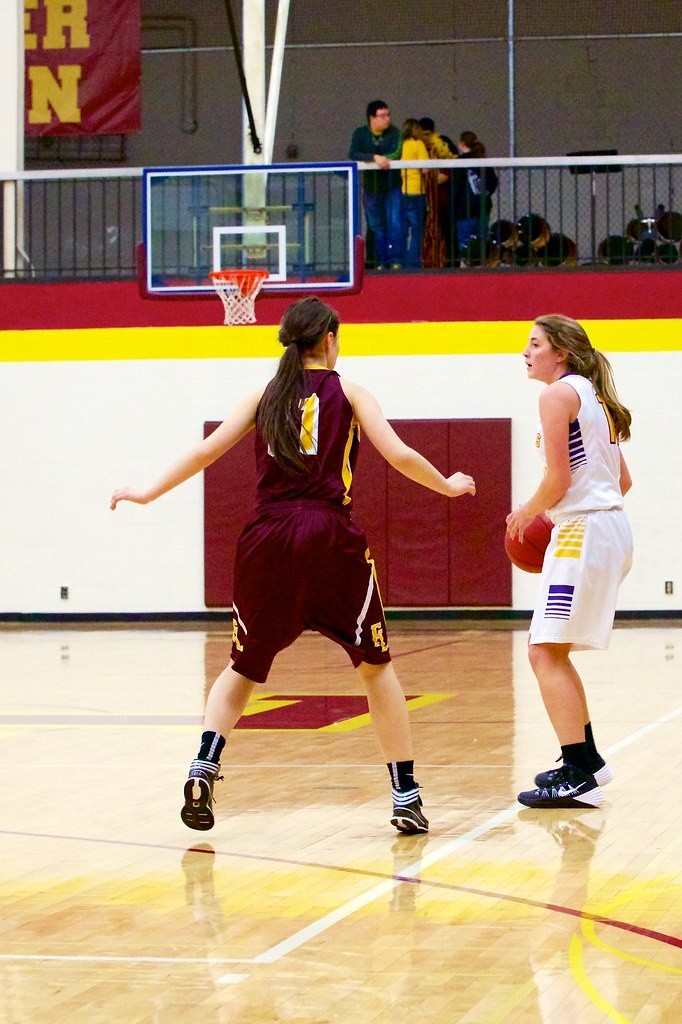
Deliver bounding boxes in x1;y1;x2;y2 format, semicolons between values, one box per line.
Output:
390;264;401;271
374;265;385;271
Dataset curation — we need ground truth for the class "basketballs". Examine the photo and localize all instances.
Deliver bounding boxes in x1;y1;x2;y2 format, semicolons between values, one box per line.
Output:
504;510;556;574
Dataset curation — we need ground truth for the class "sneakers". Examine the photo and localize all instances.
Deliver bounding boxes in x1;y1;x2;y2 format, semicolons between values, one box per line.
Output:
390;783;429;833
180;759;223;831
517;762;605;808
535;754;613;786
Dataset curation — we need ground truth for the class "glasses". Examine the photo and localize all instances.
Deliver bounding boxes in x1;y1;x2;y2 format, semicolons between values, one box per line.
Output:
374;113;390;119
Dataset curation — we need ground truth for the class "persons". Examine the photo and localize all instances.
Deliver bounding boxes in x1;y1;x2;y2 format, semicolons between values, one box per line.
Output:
110;298;476;834
349;100;497;271
504;314;632;809
